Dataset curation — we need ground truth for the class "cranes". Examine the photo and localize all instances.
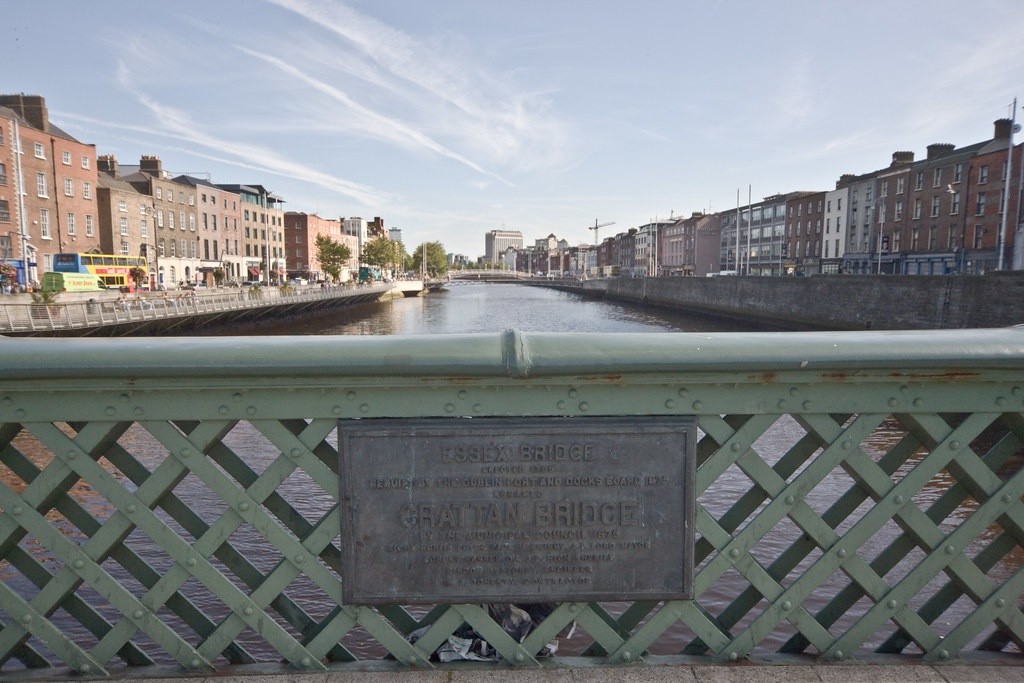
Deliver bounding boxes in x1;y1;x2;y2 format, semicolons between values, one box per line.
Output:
587;217;617;246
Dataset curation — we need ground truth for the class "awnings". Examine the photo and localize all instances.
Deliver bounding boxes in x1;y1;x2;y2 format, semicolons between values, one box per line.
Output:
249;267;289;275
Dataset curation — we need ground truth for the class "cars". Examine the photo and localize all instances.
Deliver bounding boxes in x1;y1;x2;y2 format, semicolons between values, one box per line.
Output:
183;282;206;291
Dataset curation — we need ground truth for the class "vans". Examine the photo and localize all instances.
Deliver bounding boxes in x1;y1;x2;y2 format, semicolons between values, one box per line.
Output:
42;271;111;293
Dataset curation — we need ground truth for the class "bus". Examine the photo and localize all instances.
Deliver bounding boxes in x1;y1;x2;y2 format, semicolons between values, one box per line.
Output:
53;252;151;292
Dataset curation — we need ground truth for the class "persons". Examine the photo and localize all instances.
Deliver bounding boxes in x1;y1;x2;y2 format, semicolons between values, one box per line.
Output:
114;279;373;311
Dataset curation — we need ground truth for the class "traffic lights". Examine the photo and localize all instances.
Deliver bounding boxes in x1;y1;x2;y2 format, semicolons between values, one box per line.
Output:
140;244;148;257
